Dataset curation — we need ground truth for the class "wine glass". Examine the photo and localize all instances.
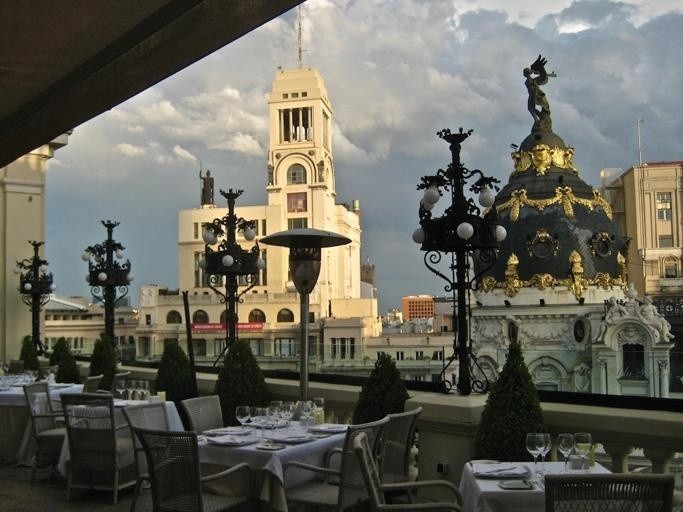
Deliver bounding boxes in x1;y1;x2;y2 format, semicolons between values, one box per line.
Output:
559;432;593;477
114;379;150;402
527;432;551;478
2;370;52;389
236;398;325;442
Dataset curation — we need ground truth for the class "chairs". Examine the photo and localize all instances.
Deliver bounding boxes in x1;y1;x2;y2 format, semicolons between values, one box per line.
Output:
8;363;174;512
353;432;464;512
131;425;255;511
283;406;424;511
544;472;674;512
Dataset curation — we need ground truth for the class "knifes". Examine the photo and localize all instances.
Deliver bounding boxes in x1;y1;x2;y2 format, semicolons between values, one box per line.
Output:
486;467;516;474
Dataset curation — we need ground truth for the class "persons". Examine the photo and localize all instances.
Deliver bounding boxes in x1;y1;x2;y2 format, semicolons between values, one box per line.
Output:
523;68;550;125
198;169;214;206
592;296;627;343
638;294;675;343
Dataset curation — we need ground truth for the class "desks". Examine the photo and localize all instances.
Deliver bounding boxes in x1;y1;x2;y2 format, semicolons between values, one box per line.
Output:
463;458;610;511
178;420;349;511
